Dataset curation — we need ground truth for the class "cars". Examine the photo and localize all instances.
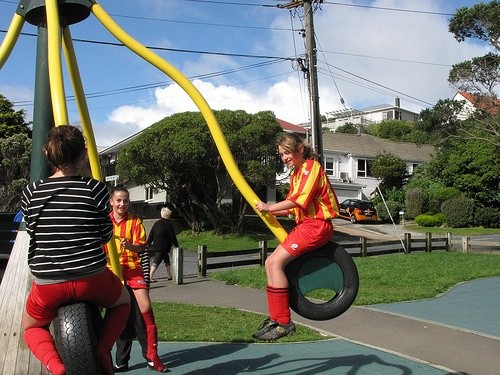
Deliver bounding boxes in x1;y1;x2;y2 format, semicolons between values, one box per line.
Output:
335;199;378;224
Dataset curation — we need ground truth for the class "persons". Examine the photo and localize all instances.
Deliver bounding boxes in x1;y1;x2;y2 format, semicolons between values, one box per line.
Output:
147;206;180;283
100;186;167;375
252;133;340;343
20;125;132;375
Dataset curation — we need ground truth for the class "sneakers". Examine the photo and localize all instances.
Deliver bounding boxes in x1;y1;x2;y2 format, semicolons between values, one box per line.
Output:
112;364;129;372
252;317;279;339
145;358;155;370
258;319;297;342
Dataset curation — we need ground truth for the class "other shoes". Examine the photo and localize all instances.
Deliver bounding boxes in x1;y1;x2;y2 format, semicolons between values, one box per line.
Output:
147;355;167;372
149;279;158;283
168;277;172;280
